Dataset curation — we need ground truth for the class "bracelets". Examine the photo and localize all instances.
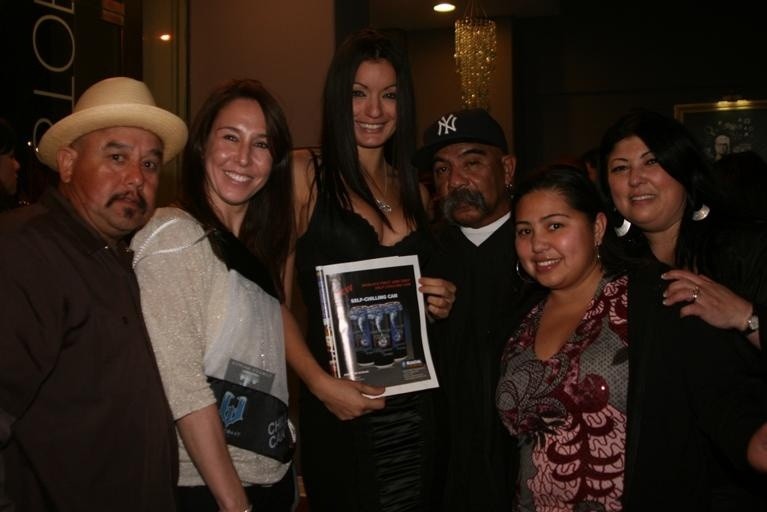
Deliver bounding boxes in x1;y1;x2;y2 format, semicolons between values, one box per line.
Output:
242;503;253;512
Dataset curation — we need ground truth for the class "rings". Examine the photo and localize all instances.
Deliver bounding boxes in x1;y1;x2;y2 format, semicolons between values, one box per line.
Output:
689;284;700;302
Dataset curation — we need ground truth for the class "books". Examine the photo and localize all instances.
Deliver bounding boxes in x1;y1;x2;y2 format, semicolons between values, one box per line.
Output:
312;251;442;401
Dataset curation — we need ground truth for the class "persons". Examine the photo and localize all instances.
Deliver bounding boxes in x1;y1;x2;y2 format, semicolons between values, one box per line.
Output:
124;75;302;512
0;117;21;212
713;135;730;160
410;108;545;512
584;149;600;184
1;72;190;511
595;105;763;367
272;24;458;511
490;164;766;511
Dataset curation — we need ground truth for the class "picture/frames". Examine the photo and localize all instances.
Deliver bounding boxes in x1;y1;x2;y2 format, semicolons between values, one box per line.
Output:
669;98;767;164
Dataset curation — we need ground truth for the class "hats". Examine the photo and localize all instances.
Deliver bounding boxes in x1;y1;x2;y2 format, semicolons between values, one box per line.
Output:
36;76;188;172
421;110;509;164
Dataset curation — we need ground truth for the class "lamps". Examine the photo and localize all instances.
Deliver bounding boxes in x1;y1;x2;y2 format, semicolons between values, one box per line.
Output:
449;1;499;115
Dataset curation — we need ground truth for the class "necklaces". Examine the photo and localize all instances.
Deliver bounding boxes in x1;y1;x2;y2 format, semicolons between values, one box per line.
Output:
358;150;391;216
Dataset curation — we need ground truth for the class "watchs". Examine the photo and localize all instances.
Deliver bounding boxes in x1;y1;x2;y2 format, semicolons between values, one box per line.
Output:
739;304;759;337
